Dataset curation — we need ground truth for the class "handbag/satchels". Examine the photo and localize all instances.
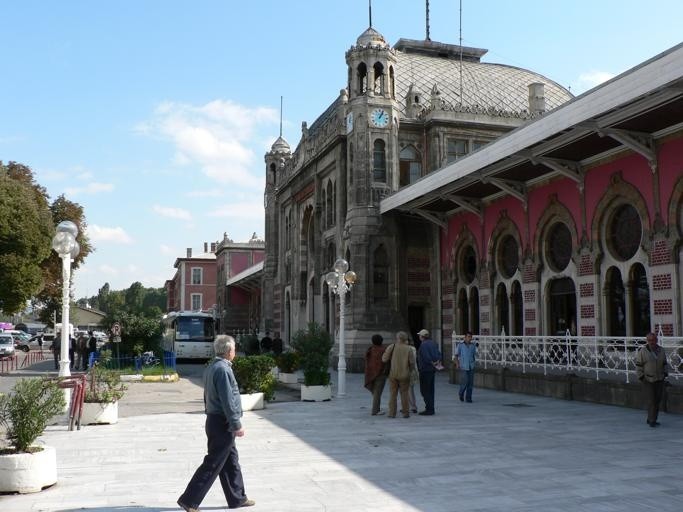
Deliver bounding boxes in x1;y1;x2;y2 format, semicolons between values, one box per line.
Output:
381;358;392;376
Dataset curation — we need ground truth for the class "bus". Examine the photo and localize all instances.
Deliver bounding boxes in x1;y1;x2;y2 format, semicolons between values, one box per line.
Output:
158;311;215;362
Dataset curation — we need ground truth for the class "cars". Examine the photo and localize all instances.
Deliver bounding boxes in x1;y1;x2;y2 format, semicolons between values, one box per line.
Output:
0;328;31;361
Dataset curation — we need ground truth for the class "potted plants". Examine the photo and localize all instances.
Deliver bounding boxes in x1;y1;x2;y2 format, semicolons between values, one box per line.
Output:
73;349;129;429
1;370;61;497
277;319;336;403
229;353;280;413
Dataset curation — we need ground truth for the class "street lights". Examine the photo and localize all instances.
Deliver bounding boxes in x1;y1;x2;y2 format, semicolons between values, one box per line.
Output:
325;258;357;396
51;220;80;412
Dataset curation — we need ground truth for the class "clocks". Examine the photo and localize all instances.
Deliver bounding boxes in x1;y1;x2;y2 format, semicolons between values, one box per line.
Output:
368;107;390;128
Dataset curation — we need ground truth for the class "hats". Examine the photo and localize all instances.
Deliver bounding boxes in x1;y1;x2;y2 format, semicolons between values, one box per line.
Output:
417;329;430;338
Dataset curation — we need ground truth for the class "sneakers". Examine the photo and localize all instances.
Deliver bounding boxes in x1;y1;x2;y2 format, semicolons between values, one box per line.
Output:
240;500;255;506
377;411;385;415
419;410;434;415
177;500;201;512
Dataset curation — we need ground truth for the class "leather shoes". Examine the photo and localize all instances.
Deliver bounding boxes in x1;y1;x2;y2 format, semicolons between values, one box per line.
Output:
459;393;464;401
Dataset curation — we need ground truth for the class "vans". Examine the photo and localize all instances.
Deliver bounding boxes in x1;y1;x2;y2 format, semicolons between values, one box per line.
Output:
21;333;55;352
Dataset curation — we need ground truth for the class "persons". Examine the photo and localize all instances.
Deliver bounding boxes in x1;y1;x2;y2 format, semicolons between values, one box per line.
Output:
361;327;445;419
48;329;284;372
635;332;668;428
452;331;478;403
176;333;255;511
